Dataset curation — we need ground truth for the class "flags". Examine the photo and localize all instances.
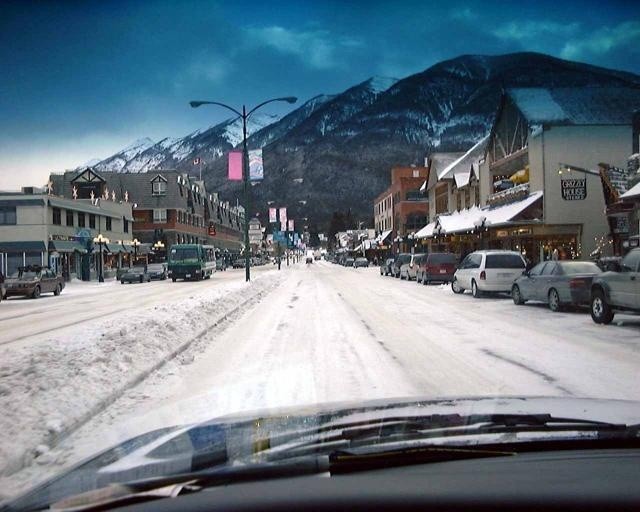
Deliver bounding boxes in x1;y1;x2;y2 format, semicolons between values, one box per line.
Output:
268;207;277;223
279;207;287;222
288;219;295;232
288;232;306;250
227;151;244;181
280;222;287;232
247;148;265;182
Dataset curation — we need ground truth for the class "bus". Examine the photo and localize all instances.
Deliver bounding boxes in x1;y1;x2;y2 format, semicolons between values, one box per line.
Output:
166;244;216;281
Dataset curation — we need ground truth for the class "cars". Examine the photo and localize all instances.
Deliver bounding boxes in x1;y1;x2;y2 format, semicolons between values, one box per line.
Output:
380;257;395;276
217;259;226;271
328;255;368;268
3;265;64;297
232;253;264;267
120;261;168;283
306;258;312;263
511;259;603;311
451;250;527;296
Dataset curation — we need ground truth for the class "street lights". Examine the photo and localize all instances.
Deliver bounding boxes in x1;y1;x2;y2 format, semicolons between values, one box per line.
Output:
93;233;109;282
251;176;310;270
130;238;140;261
191;96;299;281
155;240;164;260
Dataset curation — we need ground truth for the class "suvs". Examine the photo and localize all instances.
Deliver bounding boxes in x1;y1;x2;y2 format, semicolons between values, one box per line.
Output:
390;252;458;285
589;247;640;324
